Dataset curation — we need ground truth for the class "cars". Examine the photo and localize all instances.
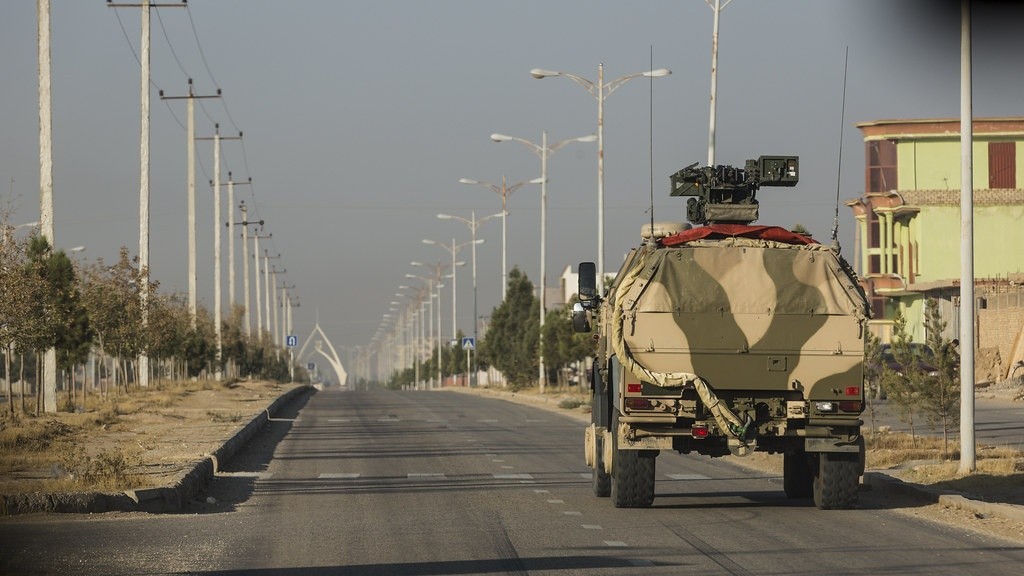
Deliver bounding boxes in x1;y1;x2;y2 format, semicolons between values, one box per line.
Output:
864;342;941;400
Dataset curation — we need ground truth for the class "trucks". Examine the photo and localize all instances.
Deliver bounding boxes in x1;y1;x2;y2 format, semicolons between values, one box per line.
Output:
571;156;874;509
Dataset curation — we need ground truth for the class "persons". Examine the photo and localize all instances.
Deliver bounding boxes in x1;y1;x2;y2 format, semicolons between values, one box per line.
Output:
942;339;960;381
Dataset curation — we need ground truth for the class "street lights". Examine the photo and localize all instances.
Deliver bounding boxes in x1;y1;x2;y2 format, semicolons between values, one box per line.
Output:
360;271;454;393
458;175;550;387
410;261;467;389
490;132;598;396
436;211;510;388
531;62;672;332
422;237;485;387
